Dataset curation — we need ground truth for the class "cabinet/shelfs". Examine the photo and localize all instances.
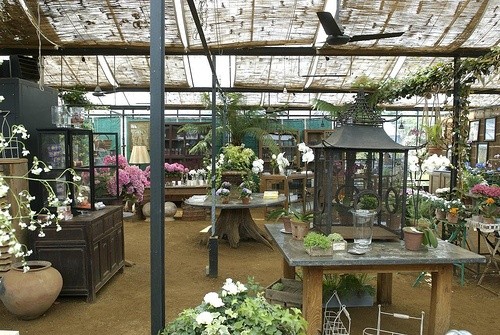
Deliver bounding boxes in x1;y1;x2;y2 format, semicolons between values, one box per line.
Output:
31;206;125;304
259;128;299;171
304;129;346;205
35;129;119;214
164;122;213;183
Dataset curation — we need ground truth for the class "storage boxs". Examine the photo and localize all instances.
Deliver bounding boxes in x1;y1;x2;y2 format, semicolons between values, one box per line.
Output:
265;277;304;305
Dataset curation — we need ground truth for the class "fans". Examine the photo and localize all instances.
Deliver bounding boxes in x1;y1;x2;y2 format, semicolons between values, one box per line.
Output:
315;11;406;46
473;214;496;227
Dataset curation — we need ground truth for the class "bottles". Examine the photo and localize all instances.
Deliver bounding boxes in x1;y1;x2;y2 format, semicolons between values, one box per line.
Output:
0;261;63;321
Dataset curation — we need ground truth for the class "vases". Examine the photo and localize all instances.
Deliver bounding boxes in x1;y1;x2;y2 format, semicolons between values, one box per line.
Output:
220;196;229;203
447;211;460;223
434;207;445;219
242;198;250;204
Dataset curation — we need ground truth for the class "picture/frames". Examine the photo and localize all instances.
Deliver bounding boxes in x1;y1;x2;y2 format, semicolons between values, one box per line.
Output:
468;115;497;167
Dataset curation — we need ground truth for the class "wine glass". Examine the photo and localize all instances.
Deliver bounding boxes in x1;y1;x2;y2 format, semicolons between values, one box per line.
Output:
351;209;377;249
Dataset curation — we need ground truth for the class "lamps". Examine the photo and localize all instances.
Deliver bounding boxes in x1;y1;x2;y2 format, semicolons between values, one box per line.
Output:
129;146;151;164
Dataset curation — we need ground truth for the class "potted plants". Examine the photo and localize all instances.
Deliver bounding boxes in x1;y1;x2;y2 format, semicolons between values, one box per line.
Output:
327;232;348;252
267;209;316;241
423;124;445;157
303;231;333;257
402;218;439;251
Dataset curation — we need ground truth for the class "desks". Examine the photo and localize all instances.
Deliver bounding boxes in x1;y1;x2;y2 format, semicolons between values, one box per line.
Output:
262;222;487;334
259;174;316;214
184;192;287;252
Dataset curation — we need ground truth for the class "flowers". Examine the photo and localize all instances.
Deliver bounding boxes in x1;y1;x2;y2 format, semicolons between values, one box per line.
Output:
239;188;253;195
0;94;86;273
215;182;230;195
161;276;306;335
407;153;500;213
82;141;315;205
